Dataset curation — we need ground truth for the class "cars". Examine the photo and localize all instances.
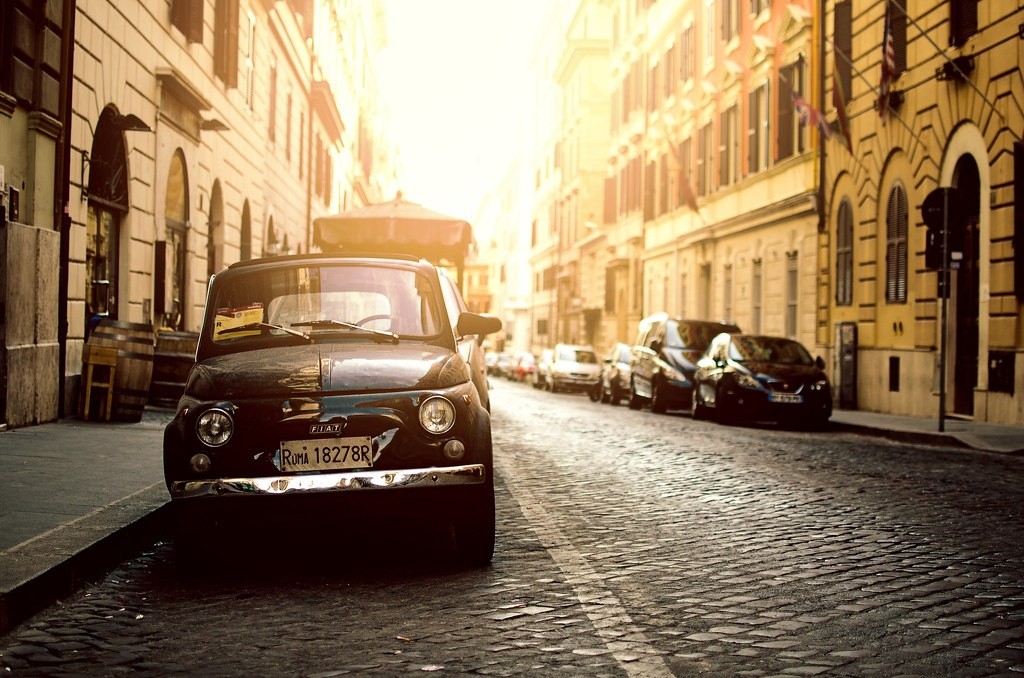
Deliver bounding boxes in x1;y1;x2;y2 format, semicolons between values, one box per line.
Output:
691;333;834;430
269;266;504;414
597;341;632;407
161;249;496;568
487;343;602;396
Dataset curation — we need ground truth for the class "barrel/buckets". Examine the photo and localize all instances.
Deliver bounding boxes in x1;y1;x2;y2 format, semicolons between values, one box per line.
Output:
80;318;155;422
148;330;200;406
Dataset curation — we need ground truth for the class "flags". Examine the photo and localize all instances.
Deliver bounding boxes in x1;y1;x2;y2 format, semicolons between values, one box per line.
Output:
882;8;896;77
675;173;699;211
833;62;854;156
794;99;833;142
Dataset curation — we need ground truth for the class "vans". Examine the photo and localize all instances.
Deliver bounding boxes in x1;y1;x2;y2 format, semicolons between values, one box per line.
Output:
627;311;744;417
